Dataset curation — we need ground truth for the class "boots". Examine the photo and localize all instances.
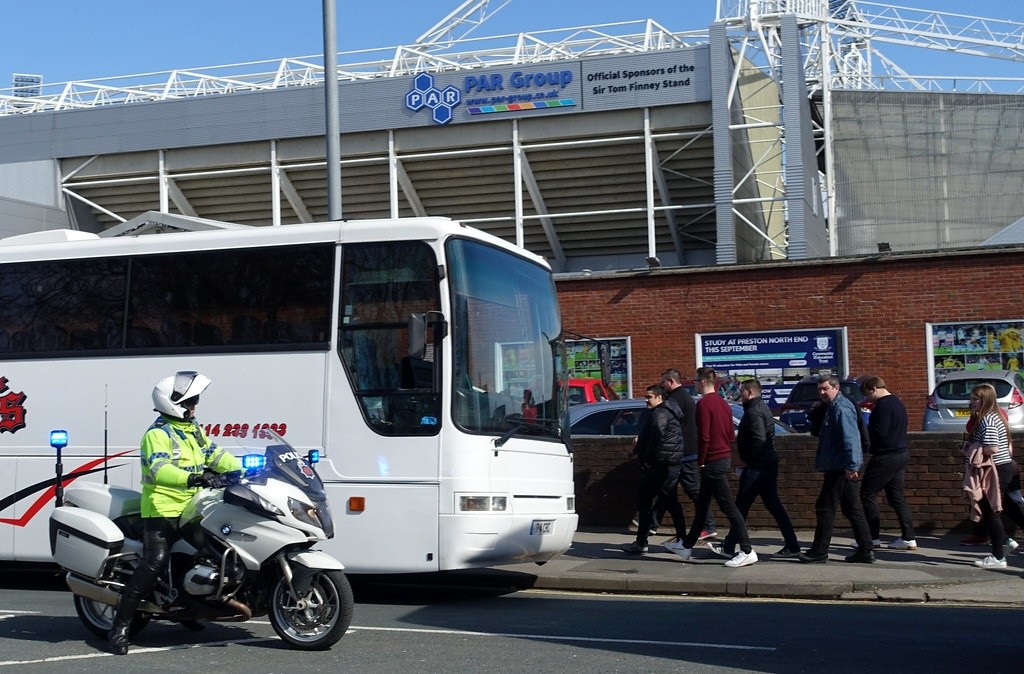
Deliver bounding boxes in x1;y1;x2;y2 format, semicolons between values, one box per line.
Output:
178;616;205;630
107;541;168;654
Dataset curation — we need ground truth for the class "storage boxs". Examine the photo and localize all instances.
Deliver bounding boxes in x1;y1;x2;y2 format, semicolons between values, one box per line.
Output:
48;506;125;580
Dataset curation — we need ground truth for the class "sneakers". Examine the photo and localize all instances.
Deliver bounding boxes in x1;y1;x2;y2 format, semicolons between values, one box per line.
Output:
658;536;677;547
697;526;718;540
960;534;991;546
632;520;657;535
845;549;875;563
724;549;758;567
620;540;648;555
664;538;692;560
888;537;917;550
797;548;828;563
1003;538;1019;557
770;546;802;561
974;555;1008;568
707;542;733;558
851;539;880;548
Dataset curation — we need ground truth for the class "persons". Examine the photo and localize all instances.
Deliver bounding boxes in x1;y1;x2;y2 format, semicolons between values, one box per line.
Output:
521;390;535;416
664;364;758;567
933;326;1022;382
507;344;531;367
851;374;919;551
707;380;801;558
618;367;717;558
960;383;1024;568
615;343;623;355
108;371;265;656
797;373;876;565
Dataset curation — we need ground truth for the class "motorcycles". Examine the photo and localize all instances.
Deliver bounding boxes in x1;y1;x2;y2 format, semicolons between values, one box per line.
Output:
50;385;354;651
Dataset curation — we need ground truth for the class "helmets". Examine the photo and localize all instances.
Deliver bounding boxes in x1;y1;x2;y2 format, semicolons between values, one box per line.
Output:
153;370;211;419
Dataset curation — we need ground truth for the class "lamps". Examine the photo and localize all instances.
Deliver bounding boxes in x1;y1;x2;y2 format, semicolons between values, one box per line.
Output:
878;242;891;253
646;256;661;267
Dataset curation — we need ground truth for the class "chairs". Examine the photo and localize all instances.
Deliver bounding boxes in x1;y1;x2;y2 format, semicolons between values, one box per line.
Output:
947;383;970;400
0;315;412;415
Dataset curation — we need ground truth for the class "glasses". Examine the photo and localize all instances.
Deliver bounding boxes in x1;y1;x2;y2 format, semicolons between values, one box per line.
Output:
185;395;200;407
692;379;701;384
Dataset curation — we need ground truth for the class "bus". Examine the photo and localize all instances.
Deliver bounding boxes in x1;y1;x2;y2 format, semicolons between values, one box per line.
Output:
0;219;613;575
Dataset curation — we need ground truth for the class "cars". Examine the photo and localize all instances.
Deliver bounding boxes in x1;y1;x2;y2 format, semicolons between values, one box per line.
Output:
517;376;622;409
921;370;1024;430
778;375;876;434
565;396;799;436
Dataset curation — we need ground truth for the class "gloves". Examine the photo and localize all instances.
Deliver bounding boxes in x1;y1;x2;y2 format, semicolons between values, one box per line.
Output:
188;473;220;488
